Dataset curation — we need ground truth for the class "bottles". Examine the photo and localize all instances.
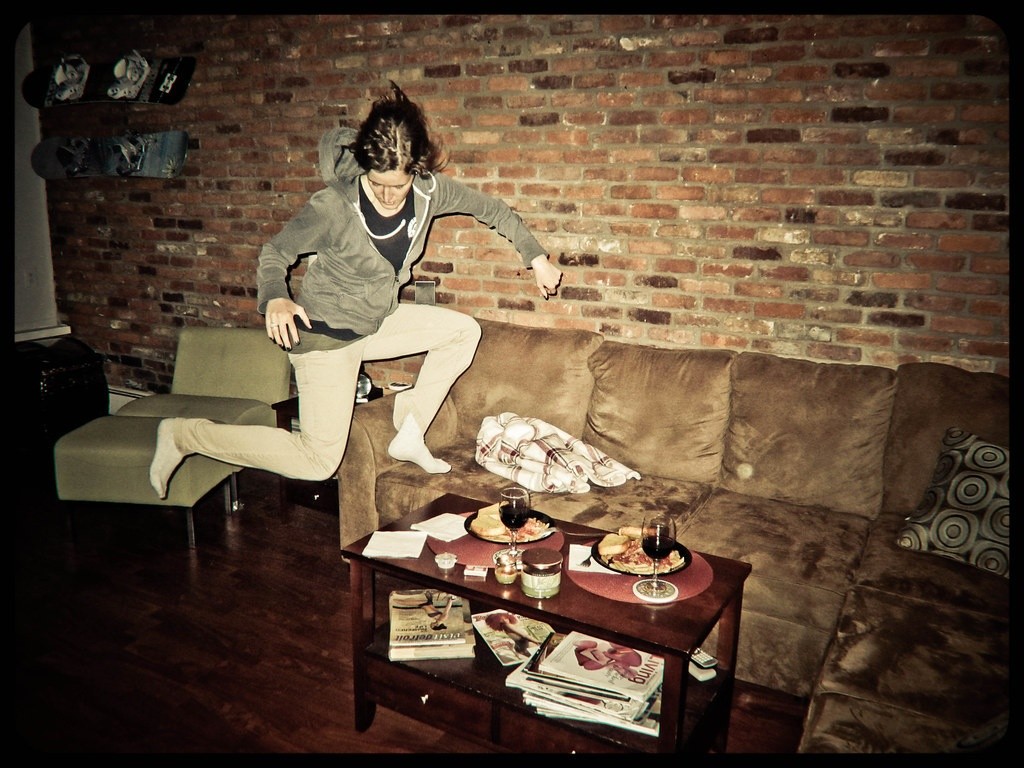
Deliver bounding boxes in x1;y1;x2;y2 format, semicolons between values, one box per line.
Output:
494;555;518;585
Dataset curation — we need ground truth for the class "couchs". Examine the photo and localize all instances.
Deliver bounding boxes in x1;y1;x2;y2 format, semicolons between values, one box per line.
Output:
54;326;291;547
336;318;1024;754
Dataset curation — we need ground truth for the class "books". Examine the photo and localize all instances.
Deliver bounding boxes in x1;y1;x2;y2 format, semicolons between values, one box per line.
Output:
387;589;476;662
471;609;665;737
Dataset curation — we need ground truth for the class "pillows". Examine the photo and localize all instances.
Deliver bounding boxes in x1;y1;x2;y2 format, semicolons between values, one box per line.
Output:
896;426;1010;579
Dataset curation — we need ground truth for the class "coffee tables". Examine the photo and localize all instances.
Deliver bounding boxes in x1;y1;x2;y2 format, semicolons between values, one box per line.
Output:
341;491;753;754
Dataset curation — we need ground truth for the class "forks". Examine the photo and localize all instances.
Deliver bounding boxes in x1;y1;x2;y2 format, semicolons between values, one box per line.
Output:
579;555;592;567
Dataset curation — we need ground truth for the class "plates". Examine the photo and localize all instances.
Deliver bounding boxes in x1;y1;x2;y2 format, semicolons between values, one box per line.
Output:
591;537;692;577
465;508;556;543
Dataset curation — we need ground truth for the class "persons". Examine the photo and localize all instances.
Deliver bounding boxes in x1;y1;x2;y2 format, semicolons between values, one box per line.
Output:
149;78;564;500
485;613;542;657
393;592;456;631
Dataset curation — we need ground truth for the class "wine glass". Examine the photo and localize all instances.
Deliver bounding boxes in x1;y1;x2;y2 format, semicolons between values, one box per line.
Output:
637;512;676;598
493;487;531;566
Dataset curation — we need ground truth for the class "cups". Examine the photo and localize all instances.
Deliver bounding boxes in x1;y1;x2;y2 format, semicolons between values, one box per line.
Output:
521;548;563;599
435;553;457;575
